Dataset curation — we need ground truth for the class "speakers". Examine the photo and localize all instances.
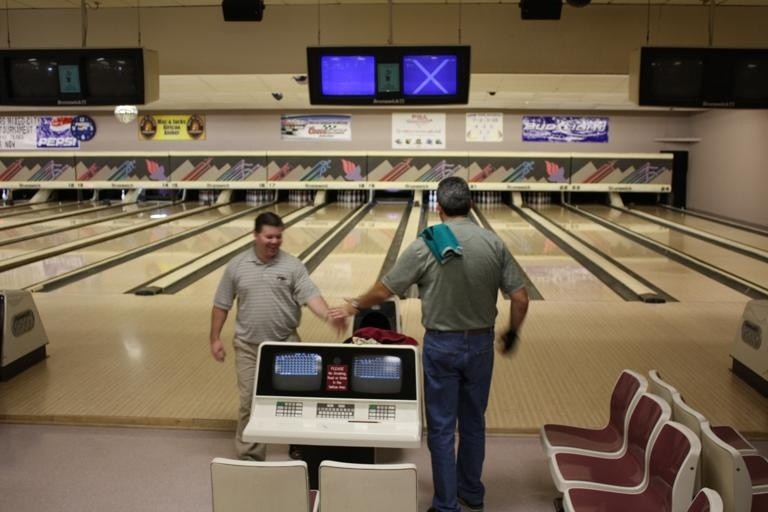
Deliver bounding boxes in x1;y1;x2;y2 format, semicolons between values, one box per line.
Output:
222;0;263;21
521;0;562;20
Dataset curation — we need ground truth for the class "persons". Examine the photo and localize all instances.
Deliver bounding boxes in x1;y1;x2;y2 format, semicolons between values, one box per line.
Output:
329;176;528;512
210;212;348;462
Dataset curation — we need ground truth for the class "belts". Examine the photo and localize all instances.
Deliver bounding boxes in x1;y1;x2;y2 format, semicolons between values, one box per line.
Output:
426;326;494;335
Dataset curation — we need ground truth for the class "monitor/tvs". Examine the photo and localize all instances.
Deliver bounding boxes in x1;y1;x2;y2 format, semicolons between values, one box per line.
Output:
255;345;417;400
629;46;768;109
306;46;471;105
0;48;159;106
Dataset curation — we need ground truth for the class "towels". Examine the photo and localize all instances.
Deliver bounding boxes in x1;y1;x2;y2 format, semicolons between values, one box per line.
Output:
344;327;419;345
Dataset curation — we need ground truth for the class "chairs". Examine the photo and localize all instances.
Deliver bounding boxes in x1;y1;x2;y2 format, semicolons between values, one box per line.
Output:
208;457;319;512
318;460;417;512
538;370;768;512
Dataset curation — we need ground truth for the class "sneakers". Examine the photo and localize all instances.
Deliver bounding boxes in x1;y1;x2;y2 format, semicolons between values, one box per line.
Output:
427;496;483;512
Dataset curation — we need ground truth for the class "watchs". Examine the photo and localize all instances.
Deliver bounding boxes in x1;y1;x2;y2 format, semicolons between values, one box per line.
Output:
352;298;367;313
